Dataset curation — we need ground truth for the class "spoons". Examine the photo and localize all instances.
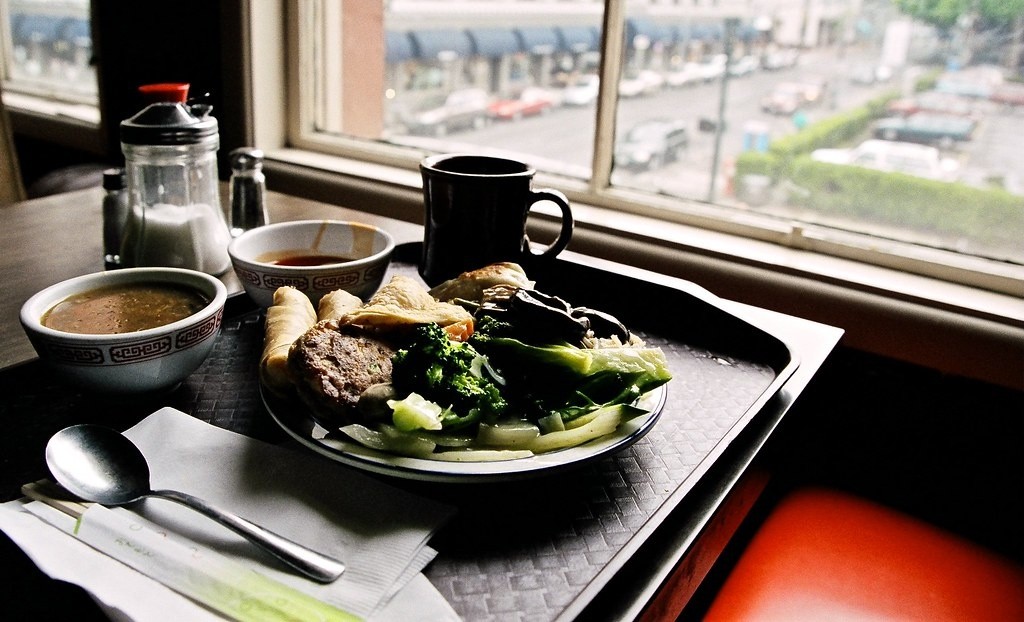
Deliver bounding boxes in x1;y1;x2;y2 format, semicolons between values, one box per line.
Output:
47;427;346;583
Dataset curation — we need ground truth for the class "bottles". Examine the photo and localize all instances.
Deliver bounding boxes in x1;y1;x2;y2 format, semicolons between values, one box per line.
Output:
121;84;233;276
101;171;133;262
228;147;267;233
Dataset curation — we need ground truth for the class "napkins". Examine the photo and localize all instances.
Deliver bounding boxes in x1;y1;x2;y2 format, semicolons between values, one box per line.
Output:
0;407;461;622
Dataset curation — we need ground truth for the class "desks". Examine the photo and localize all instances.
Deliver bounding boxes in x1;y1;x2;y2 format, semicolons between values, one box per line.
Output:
0;180;846;622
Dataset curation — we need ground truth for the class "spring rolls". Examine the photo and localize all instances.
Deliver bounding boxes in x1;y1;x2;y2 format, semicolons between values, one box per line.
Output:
260;285;318;396
317;289;362;325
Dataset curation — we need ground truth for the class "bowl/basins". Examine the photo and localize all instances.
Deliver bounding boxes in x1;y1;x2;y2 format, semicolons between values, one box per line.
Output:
22;268;227;392
228;221;396;309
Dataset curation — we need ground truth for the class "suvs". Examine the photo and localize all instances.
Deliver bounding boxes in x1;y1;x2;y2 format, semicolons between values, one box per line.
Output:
612;118;691;172
400;87;496;136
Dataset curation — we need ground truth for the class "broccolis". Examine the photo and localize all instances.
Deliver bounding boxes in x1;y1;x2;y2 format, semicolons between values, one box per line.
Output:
471;314;592;374
391;321;508;428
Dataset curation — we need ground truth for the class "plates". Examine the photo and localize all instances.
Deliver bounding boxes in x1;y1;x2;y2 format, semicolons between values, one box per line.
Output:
258;359;667;483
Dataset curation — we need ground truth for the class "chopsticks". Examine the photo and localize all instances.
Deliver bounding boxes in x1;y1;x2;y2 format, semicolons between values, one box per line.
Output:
23;479;90;519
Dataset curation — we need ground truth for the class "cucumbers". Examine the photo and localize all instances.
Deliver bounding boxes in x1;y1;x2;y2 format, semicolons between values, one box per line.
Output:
466;317;672;448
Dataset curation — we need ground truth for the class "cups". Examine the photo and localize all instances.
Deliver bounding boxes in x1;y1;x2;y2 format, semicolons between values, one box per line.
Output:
417;155;571;287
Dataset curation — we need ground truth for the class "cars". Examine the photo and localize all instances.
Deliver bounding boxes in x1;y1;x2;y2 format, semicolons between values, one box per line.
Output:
488;43;798;119
807;72;1023;182
757;81;826;113
